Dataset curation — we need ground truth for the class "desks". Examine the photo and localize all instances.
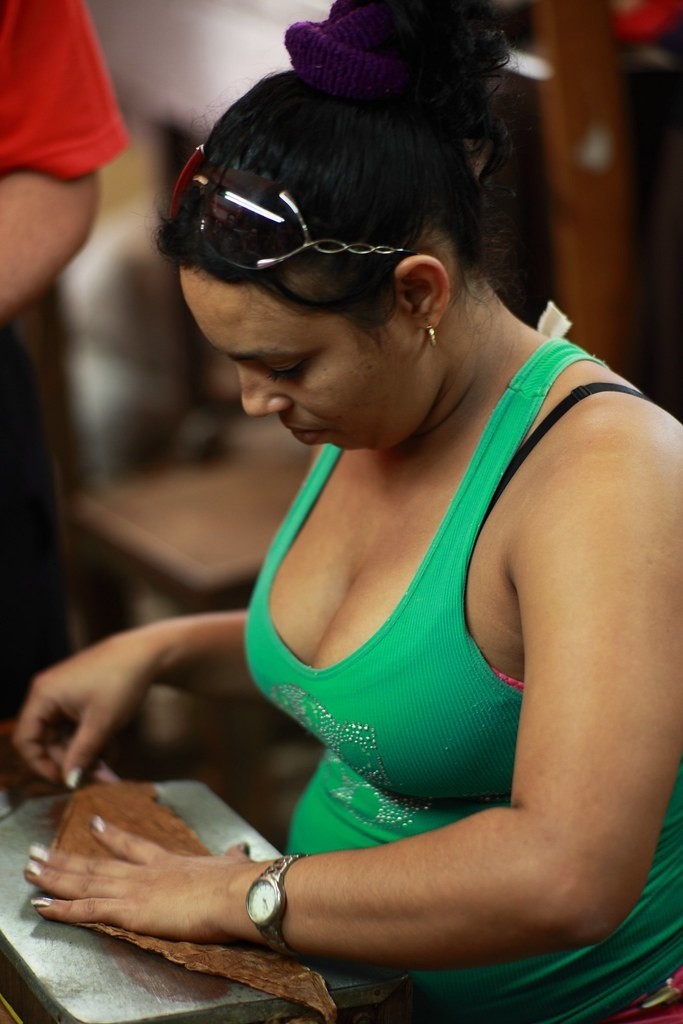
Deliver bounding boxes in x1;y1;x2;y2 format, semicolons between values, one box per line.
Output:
0;719;413;1024
65;431;312;768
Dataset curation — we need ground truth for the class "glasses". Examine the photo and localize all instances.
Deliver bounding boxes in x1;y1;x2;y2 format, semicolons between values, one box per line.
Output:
157;140;422;268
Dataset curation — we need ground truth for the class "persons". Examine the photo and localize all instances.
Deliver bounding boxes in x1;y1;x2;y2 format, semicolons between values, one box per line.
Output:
12;0;683;1024
0;0;131;719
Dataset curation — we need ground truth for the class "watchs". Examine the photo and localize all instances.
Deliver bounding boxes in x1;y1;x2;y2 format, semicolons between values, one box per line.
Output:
246;853;313;959
